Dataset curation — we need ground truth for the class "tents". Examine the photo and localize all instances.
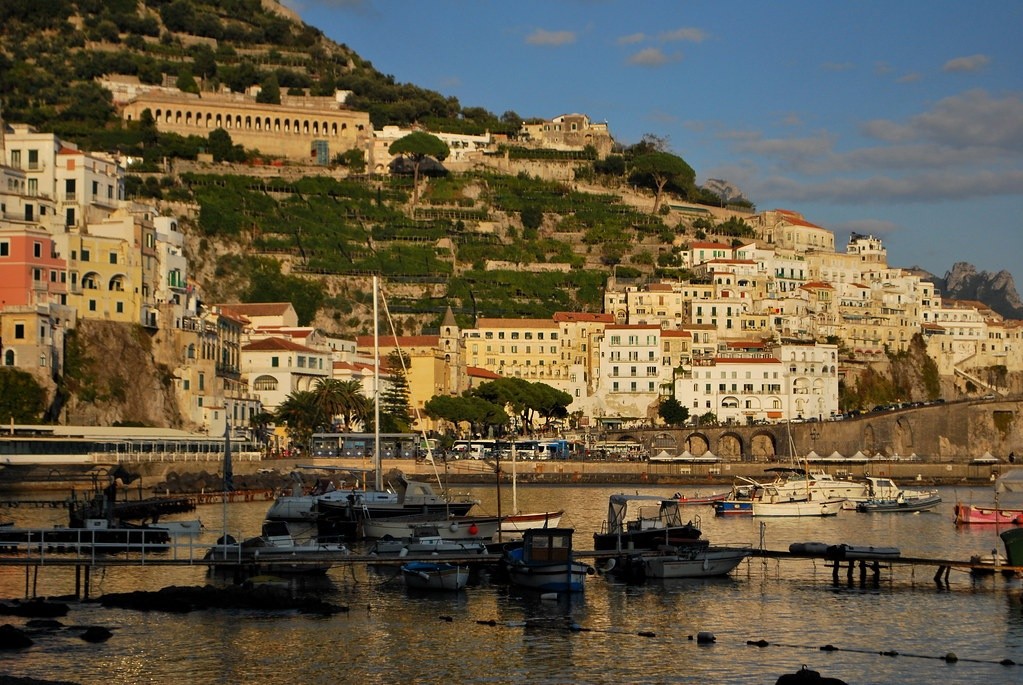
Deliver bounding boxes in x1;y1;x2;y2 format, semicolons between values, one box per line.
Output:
905;453;922;461
694;450;722;461
870;452;887;461
887;452;904;460
846;451;869;460
798;451;822;460
974;451;998;463
822;451;845;460
672;451;696;460
649;450;672;460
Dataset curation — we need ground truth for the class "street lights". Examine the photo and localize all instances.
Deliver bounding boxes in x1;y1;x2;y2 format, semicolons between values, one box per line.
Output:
990;470;999;536
810;428;820;451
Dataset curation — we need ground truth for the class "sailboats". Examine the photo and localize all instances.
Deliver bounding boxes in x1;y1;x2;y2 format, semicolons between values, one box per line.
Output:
264;275;476;521
498;444;564;531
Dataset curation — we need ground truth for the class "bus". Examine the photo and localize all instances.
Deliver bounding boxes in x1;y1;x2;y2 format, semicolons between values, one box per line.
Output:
594;444;642;457
452;440;538;459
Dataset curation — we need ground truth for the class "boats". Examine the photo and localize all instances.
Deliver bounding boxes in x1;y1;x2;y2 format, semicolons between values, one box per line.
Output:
499;528;591;592
362;513;508;542
752;461;848;517
656;492;730;506
370;525;488;558
855;496;943;513
203;521;345;575
712;484;812;517
736;468;932;501
970;548;1008;564
953;501;1023;525
401;561;470;591
593;494;753;580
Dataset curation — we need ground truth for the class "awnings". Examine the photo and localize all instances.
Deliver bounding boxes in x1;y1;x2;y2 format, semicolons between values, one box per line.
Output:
767;412;782;418
600;419;622;424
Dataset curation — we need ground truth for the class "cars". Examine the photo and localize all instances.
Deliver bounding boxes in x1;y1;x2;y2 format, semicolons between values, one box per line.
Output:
685;422;697;427
848;399;945;418
538;443;558;454
983;394;995;400
828;414;843;421
452;445;485;460
